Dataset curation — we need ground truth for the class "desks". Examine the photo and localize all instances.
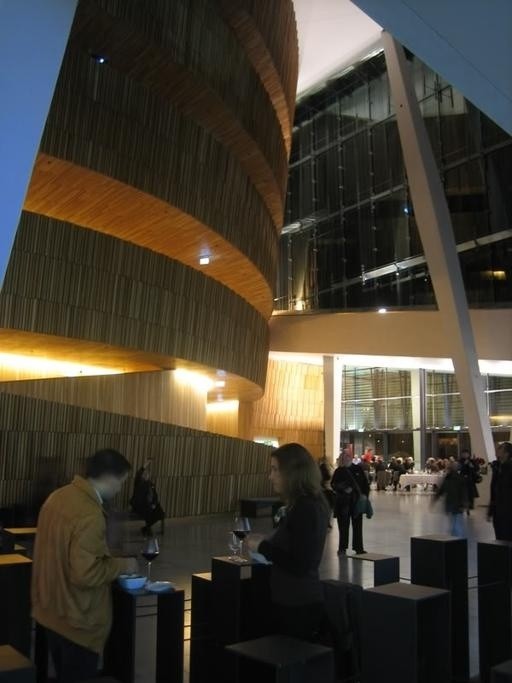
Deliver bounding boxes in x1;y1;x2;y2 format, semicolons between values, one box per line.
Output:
397;473;443;494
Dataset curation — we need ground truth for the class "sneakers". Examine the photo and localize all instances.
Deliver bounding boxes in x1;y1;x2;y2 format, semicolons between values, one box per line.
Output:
141;526;153;538
338;547;366;555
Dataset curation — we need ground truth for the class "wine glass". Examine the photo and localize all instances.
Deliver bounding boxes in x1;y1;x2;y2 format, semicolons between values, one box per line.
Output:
232;517;251;563
228;532;240;560
140;536;160;585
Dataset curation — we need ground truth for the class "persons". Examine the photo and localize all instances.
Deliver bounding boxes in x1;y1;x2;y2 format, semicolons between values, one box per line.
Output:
31;447;140;682
318;442;512;556
134;472;164;537
241;442;331;645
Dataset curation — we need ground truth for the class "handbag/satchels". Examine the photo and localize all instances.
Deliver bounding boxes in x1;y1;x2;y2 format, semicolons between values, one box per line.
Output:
354;493;367;513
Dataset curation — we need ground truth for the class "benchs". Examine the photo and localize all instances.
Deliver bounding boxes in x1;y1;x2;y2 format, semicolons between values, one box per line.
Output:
236;497;284;517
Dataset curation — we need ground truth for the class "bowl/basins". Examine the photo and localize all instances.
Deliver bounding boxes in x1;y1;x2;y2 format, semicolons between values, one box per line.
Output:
119;574;148;590
